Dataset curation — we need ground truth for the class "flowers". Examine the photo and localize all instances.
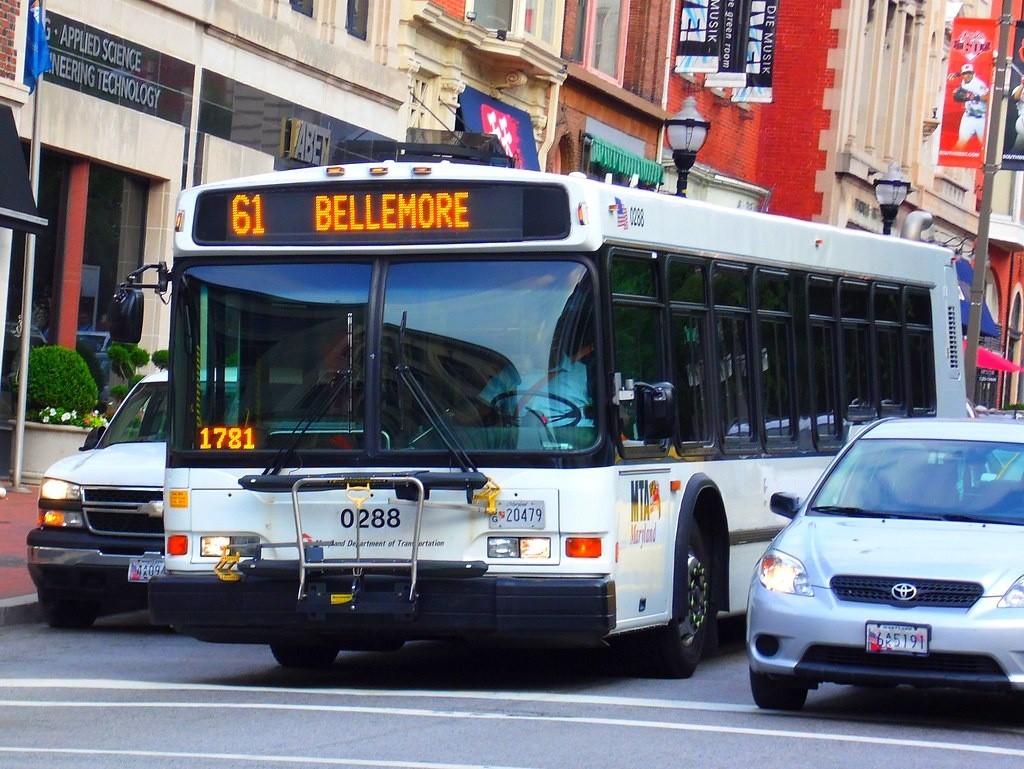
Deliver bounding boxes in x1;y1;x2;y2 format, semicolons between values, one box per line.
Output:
38;406;109;429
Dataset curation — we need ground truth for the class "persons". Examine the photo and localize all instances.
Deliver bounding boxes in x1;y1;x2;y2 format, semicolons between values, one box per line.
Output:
443;318;596;427
1010;74;1024;154
953;63;990;149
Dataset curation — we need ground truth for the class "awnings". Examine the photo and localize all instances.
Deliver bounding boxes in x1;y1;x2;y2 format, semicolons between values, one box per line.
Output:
919;239;1000;338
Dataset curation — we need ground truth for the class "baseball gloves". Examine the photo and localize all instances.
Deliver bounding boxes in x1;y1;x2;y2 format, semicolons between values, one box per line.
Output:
952;86;975;103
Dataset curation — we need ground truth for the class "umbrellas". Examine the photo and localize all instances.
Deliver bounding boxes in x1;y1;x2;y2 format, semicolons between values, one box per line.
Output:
963;339;1024;419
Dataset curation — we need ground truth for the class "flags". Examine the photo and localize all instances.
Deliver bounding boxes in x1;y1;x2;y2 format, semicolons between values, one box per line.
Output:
23;0;53;96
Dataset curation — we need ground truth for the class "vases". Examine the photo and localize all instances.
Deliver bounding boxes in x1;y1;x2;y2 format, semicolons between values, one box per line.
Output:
9;418;93;490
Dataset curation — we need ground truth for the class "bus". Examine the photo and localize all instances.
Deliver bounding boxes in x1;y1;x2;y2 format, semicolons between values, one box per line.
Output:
108;148;980;679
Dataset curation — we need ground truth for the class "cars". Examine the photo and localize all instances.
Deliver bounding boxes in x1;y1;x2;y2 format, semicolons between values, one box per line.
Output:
746;411;1024;713
23;367;323;632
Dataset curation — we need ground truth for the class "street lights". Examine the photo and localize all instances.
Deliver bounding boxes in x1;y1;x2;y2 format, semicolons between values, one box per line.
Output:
665;94;712;198
870;162;915;235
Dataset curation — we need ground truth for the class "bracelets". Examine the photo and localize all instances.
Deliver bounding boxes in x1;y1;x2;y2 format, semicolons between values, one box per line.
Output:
445;408;456;421
